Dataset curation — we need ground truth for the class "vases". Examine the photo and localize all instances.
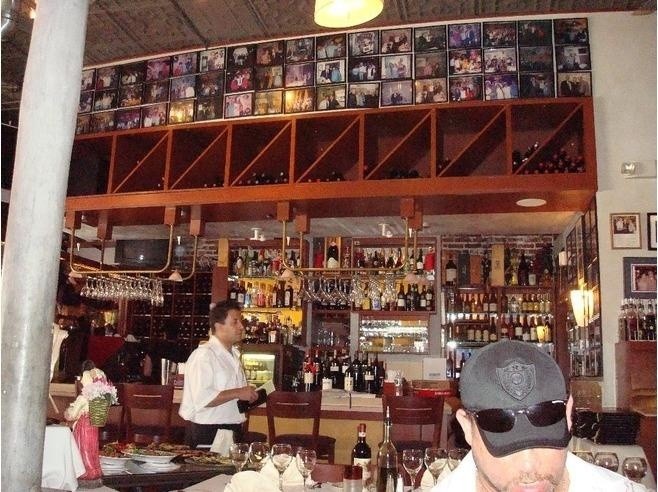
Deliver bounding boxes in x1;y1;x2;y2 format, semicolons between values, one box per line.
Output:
89;396;111;427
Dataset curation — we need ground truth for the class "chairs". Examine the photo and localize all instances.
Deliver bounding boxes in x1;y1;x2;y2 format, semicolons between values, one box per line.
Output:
374;391;446;456
571;407;597;442
99;422;118;449
587;406;641;444
238;410;267;445
119;384;186;444
264;385;337;464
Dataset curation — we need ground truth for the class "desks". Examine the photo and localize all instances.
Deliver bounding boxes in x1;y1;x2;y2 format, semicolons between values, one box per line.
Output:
38;424;86;491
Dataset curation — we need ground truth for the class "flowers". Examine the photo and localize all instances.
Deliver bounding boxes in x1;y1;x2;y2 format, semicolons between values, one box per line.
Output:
61;364;120;421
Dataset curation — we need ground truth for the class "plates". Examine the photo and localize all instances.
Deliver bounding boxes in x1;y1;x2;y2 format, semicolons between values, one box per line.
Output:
132;460;179;473
121;449;177;464
101;468;127;476
97;455;133;467
182;458;228;468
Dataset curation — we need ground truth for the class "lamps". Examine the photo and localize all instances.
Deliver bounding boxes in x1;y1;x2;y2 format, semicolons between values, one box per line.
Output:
167;267;185;283
618;159;655;180
311;0;389;31
569;285;588;329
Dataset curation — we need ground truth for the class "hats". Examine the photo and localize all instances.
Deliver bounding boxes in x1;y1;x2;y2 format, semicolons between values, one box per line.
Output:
459;338;572;457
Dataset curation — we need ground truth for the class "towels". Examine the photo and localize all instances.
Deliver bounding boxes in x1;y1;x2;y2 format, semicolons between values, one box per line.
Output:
222;467;282;492
283;450;311;486
210;428;236;455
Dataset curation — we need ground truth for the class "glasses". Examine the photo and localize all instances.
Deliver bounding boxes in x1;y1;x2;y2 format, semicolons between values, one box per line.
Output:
461;391;570;433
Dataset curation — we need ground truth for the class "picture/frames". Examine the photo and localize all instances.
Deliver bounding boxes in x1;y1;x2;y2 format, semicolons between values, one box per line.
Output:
71;15;592;134
646;211;657;253
609;211;643;250
621;255;657;299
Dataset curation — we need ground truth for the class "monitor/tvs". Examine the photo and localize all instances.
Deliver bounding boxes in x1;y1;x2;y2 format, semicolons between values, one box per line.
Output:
114;239;167;266
239;352;280;391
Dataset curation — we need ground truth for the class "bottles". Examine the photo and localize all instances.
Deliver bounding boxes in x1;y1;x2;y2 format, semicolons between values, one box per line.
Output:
377;416;398;492
617;302;657;341
317;328;334;346
231;169;345;185
438;156;450;171
384;167;419;179
317;283;434;311
460;351;472;368
637;270;657;291
480;248;493;284
444;290;552;345
301;348;383;394
312;239;434;275
518;247;530;285
202;175;223;188
228;250;298;308
130;276;213;348
510;249;519;286
446;351;456;379
394;372;403;398
351;422;372;487
246;319;296;345
513;140;585;175
444;254;459;287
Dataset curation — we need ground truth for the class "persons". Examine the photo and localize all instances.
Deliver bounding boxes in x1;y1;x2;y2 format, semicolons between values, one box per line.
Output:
171;83;195;99
422;340;651;492
419;31;433;49
451;54;481;73
176;60;193;76
353;61;376;81
399;33;407;46
453;25;474;47
319;95;339;109
454;81;479;100
177;298;259;445
320;64;342;82
154;62;166;79
127;72;136;83
486;77;518;99
434;83;442;94
490;55;513;72
424;62;442;75
227;94;251;116
208;52;222;70
609;213;657;294
199;104;210;120
145;108;166;127
103;73;110;87
559;74;590;97
391;90;398;105
317;40;337;58
231;72;253;91
348;88;376;108
143;319;186;383
151;85;161;103
388;61;406;78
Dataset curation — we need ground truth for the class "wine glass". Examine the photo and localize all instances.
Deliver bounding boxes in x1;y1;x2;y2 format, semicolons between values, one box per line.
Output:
293;448;317;491
402;449;423;492
425;447;448;485
79;273;165;308
297;279;398;307
273;444;294;491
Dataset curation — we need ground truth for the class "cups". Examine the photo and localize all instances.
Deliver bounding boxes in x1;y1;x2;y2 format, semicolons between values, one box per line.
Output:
343;465;363;491
383;335;394;352
413;337;424;353
529;274;536;286
447;449;469;473
386;472;403;492
333;336;345;348
244;370;271;381
226;442;271;472
570;451;648;484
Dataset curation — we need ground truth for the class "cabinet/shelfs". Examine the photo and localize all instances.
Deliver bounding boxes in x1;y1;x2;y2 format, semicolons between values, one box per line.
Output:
614;340;656;483
117;266;211;362
309;311;351;364
216;239;305;345
66;94;595;236
442;284;560;382
313;237;441;313
